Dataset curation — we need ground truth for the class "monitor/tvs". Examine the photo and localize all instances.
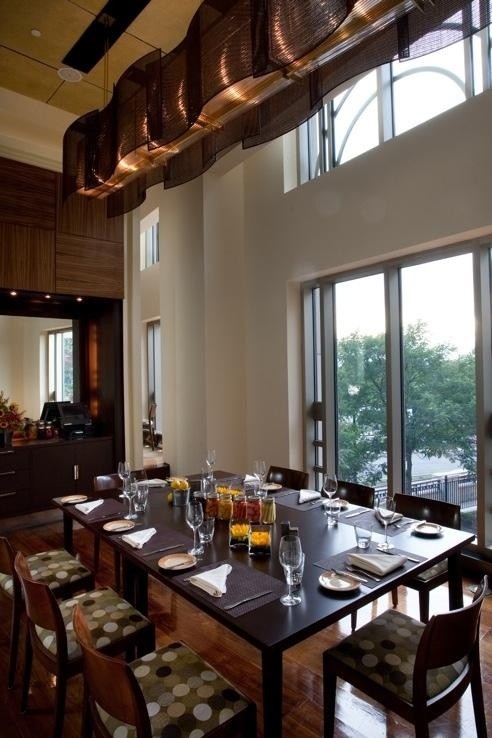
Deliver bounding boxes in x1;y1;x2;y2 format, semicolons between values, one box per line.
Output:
56;401;90;429
40;401;72;420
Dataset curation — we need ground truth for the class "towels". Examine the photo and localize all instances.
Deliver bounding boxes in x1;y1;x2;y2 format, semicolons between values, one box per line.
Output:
375;508;403;525
299;489;321;504
135;478;165;488
347;554;406;577
75;499;103;515
122;528;156;548
189;564;232;597
243;474;258;487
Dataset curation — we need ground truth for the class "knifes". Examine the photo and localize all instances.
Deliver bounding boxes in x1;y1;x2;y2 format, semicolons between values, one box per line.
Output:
375;546;422;564
221;587;275;610
346;509;371;519
143;543;185;557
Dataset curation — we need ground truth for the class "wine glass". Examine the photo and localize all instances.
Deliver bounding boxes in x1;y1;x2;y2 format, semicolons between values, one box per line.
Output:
253;459;270;496
117;462;131;499
125;478;140;518
322;474;338;515
279;537;303;604
375;498;397;549
206;448;217;477
187;503;204;554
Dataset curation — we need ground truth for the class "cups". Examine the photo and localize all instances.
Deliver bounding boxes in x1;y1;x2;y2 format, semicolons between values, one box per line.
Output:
171;487;190;505
353;520;374;549
133;479;149;512
251;525;272;556
197;518;215;543
285;554;306;586
326;505;340;528
228;519;252;549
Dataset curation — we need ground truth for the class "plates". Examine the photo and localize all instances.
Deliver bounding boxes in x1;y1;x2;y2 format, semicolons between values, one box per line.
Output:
61;495;88;504
157;554;199;571
414;520;444;535
318;571;361;593
102;520;136;532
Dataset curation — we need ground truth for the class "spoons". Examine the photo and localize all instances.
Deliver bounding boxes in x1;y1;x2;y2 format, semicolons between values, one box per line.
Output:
394;519;418;528
312;499;327;505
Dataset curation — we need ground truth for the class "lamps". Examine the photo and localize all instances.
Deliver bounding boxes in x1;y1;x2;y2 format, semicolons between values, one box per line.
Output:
64;0;491;217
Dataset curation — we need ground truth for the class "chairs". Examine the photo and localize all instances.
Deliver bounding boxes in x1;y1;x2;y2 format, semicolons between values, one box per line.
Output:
321;480;375;507
93;473;122;497
324;575;489;738
13;551;155;738
0;537;95;689
392;493;463;622
265;466;309;491
93;463;171;493
72;604;257;738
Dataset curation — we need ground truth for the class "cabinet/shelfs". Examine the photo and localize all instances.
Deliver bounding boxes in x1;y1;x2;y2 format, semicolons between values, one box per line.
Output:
32;440;114;510
1;449;32;519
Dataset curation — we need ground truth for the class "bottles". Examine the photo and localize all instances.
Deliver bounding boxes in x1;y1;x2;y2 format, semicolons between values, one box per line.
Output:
205;492;218;519
262;496;276;524
191;490;206;510
28;421;38;439
46;422;53;438
218;494;233;520
39;420;46;439
246;495;260;524
233;496;245;520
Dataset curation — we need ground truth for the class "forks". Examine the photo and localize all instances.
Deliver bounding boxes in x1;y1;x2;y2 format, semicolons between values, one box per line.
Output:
345;564;380;583
